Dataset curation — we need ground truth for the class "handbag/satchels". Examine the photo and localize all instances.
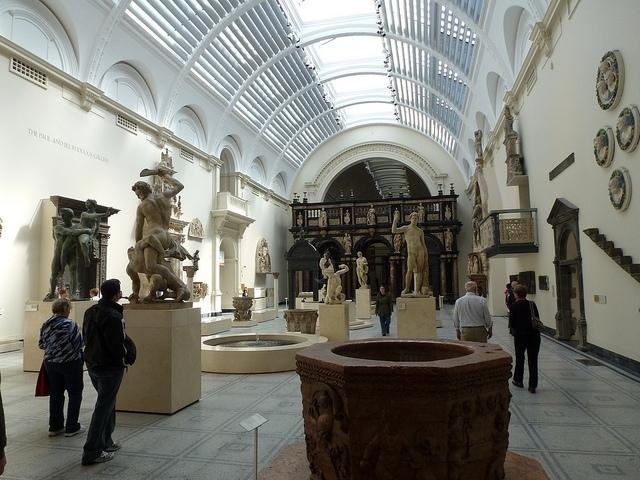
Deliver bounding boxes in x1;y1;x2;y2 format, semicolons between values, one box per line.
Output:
530;300;543;332
34;361;49;398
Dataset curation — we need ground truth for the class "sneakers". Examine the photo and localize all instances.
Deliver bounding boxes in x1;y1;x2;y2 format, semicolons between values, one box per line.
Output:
512;380;524;388
82;451;114;466
64;424;86;437
105;441;123;451
528;388;536;393
48;426;66;436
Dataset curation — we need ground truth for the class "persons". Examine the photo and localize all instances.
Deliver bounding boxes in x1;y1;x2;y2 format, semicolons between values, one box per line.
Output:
126;235;169;303
319;207;328;228
132;175;192;302
391;212;428;295
444;228;455;252
509;285;541;394
59;198;111;275
44;207;90;300
0;373;8;477
344;210;351;224
375;286;393;336
367;205;376;226
348;234;352;246
324;264;349;304
327;293;345;304
504;280;519;335
416;203;425;222
393;233;402;253
393;208;400;221
477;285;487;298
320;253;334;280
343;232;352;255
453;281;493;342
356;251;369;285
82;279;136;467
296;211;304;226
38;298;87;438
359;261;367;286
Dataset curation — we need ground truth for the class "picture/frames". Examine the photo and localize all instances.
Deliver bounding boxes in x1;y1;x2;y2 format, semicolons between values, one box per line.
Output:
606;166;632;213
595;49;625;112
592;125;614;168
614;103;640;153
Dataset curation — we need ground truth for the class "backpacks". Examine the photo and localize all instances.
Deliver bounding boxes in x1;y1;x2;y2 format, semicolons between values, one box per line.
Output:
85;303;117;369
